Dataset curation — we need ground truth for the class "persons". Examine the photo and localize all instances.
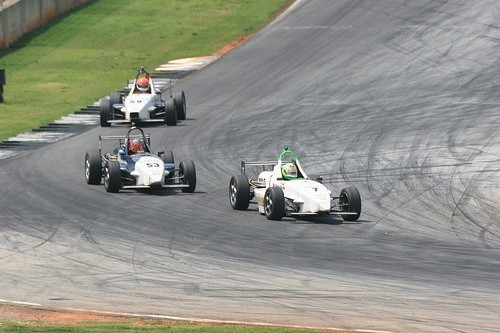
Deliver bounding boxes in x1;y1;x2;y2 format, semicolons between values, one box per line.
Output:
134;77;151;94
130;137;143;153
282;163;297;180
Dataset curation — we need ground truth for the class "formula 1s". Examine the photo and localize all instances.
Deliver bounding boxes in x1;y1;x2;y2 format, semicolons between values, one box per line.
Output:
228;145;362;221
100;64;187;127
84;126;197;194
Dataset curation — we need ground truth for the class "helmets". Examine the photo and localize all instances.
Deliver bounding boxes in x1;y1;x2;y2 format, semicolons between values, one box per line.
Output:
136;78;149;92
129;139;144;153
282;163;297;180
